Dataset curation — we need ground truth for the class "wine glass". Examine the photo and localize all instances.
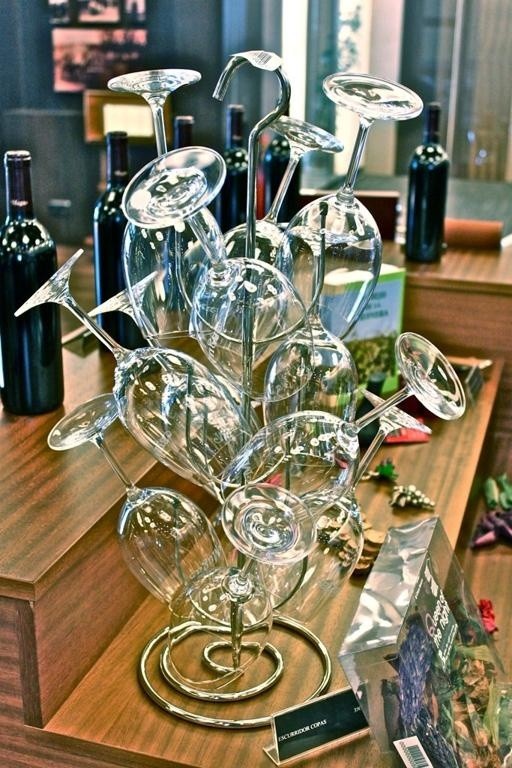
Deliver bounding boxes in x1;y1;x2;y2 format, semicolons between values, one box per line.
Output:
11;248;232;484
169;486;318;691
252;391;432;628
119;153;315;402
263;221;361;447
102;66;232;339
87;267;251;473
193;114;343;340
275;67;426;339
217;330;467;515
46;396;230;618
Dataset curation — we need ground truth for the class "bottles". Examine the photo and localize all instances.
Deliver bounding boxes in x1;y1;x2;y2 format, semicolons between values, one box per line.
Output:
264;106;300;253
219;103;254;284
92;132;157;357
0;148;64;413
165;110;212;311
406;94;451;266
